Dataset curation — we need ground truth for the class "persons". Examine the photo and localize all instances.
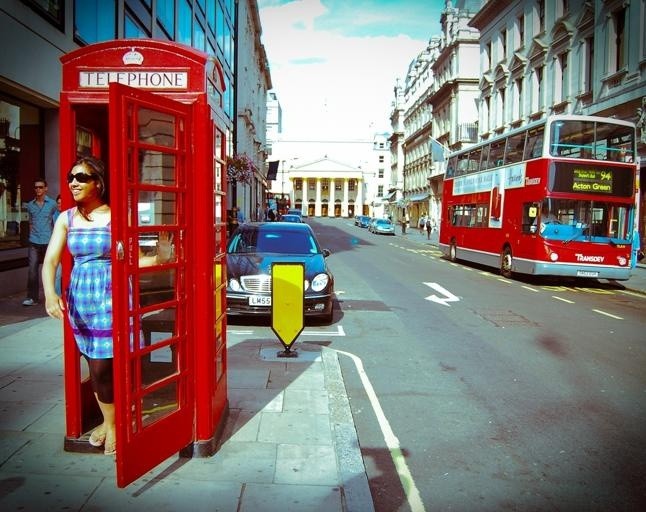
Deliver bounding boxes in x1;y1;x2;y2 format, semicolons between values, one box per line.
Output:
51;193;63;300
22;179;57;306
418;212;433;241
41;157;174;455
256;202;281;222
237;206;244;224
531;203;559;233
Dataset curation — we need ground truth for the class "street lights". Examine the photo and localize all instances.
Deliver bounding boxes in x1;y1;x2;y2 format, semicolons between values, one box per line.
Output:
282;157;299;198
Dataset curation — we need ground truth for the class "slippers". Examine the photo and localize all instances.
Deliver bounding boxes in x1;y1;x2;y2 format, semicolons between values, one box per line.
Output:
89;431;117;456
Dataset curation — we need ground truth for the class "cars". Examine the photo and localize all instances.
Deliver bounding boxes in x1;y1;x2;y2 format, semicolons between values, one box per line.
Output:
355;214;395;234
226;208;334;323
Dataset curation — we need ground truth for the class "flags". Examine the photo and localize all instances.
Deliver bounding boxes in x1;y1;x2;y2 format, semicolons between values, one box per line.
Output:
431;137;445;163
266;160;278;180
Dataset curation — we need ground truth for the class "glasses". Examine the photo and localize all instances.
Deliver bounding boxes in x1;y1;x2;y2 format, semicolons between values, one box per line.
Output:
35;185;44;189
68;172;97;183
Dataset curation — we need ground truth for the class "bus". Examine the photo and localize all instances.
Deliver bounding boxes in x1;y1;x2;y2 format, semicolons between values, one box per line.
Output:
439;115;637;281
267;192;291;215
